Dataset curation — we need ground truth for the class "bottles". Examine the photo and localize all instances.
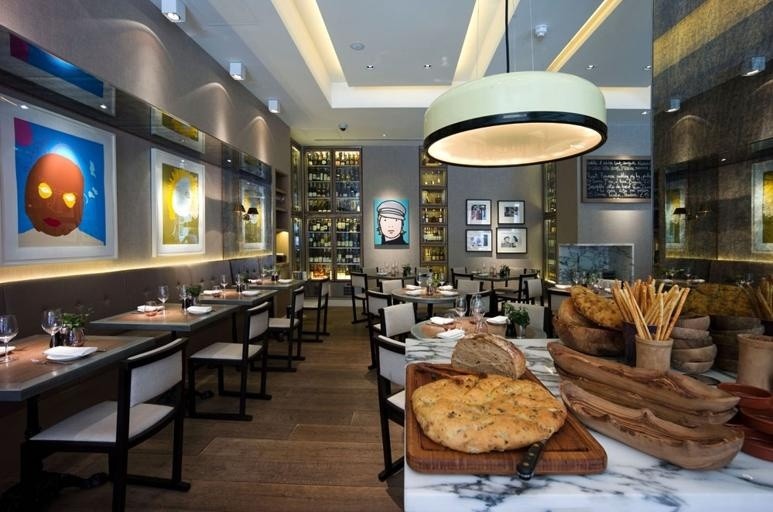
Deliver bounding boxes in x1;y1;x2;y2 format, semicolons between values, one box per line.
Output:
305;151;332;282
418;148;447;280
545;160;557;283
492;264;497;277
332;151;360;281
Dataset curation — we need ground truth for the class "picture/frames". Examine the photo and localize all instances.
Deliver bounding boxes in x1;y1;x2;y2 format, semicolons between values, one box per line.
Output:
151;147;209;257
464;198;528;255
558;241;636;283
0;93;120;263
240;178;273;250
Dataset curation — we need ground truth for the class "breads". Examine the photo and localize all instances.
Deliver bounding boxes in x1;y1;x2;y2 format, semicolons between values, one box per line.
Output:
451;332;526;379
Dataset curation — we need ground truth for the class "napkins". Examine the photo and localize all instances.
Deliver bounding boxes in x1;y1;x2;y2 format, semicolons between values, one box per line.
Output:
136;304;163;312
42;345;98;357
187;305;214;313
1;345;16;355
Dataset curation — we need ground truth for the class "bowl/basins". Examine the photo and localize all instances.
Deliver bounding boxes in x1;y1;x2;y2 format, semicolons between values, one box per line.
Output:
709;313;763;371
550;312;621;355
720;380;769;458
670;313;717;373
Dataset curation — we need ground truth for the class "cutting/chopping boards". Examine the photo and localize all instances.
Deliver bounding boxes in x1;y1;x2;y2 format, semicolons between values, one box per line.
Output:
401;359;607;475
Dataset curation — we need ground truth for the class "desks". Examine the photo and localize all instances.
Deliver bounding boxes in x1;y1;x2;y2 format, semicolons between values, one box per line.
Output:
400;332;772;511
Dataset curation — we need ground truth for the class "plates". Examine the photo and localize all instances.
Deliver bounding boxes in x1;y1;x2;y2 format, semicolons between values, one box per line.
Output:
45;345;98;361
0;345;14;358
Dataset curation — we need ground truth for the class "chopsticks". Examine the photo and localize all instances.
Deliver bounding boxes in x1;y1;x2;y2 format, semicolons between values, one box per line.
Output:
610;277;688;339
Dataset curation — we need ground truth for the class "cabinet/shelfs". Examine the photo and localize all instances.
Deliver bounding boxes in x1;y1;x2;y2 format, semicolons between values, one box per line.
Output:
417;145;448;281
300;146;362;282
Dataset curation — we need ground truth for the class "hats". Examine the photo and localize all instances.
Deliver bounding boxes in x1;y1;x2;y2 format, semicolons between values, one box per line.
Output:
375;200;406;221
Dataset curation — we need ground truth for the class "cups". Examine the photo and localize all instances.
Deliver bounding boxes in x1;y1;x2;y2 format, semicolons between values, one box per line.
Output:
443;312;456;332
623;319;659;367
635;335;673;372
144;301;158;317
570;270;601;286
465;262;493;276
736;331;772;390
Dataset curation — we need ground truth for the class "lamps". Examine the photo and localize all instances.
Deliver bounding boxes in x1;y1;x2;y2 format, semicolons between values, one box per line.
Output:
663;97;682;115
230;62;247;81
268;99;281;114
421;0;609;170
738;55;767;78
161;0;186;23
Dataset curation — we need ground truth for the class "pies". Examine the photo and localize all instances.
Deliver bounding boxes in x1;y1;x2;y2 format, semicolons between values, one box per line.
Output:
411;375;568;454
557;279;757;353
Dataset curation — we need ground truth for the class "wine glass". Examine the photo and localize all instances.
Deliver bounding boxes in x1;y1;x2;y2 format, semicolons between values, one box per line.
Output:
384;259;399;276
453;292;486;333
40;307;64;350
158;266;278;315
0;313;20;365
414;272;445;295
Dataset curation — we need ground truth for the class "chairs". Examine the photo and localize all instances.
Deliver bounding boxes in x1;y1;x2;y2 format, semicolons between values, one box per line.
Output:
20;338;192;510
186;301;272;421
286;284;330;343
252;285;305;373
346;256;771;484
